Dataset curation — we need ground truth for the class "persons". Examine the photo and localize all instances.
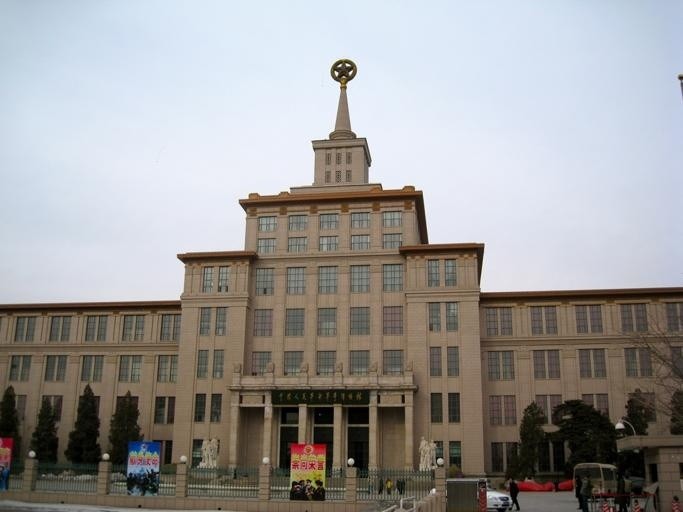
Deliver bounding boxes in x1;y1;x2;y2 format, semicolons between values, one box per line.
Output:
368;476;405;495
127;469;159;495
624;474;633;507
509;476;521;511
614;471;628;512
580;472;593;512
575;475;582;509
0;465;10;490
291;479;325;501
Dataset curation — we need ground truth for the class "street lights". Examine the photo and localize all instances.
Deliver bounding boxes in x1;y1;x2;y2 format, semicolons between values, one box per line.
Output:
615;416;637;437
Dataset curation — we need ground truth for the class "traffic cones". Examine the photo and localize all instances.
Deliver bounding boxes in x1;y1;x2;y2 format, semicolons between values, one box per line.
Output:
600;498;610;511
671;495;683;512
632;499;640;511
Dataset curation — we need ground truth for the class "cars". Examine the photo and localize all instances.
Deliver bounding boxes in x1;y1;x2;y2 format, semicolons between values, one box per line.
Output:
431;482;511;511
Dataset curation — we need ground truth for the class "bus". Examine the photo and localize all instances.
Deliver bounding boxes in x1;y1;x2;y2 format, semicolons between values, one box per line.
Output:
573;461;634;502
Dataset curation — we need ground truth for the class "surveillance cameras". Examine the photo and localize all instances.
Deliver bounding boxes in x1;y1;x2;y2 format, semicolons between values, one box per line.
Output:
616;422;625;433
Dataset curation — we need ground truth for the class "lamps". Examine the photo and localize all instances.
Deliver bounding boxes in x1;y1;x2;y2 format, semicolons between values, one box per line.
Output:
347;458;355;467
180;455;187;464
615;419;636;436
101;453;110;461
262;457;269;465
28;450;36;459
437;458;444;467
